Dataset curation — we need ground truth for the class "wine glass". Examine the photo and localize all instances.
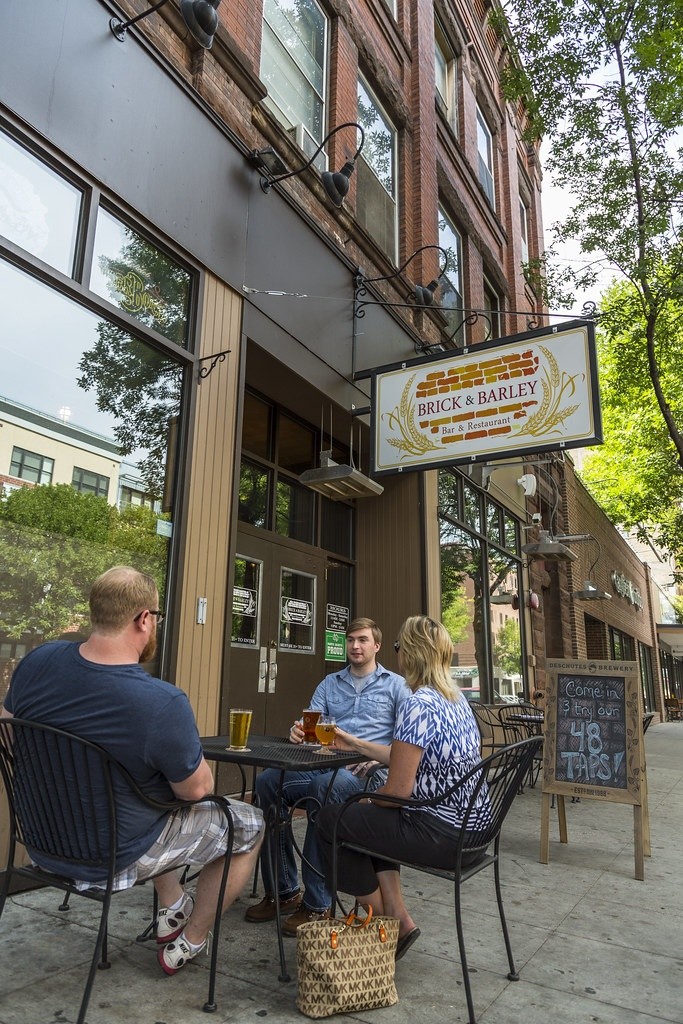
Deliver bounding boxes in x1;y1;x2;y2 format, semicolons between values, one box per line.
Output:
312;715;338;755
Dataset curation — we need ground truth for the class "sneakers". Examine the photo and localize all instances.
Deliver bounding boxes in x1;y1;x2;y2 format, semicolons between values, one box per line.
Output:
279;905;331;938
154;886;198;942
245;889;301;924
159;924;214;974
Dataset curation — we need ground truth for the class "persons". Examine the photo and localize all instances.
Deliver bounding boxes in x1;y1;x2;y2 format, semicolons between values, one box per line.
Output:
0;565;266;976
319;616;494;961
244;618;414;938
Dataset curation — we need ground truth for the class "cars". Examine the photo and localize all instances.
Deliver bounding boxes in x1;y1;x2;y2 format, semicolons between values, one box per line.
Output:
460;687;524;706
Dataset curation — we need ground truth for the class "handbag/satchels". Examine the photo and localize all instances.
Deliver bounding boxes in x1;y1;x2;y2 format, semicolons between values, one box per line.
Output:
297;903;398;1018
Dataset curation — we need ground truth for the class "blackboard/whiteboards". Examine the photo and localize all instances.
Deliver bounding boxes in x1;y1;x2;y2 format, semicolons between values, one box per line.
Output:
541;657;649;805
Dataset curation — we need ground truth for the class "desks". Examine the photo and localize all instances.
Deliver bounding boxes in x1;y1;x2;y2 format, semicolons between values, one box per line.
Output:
507;714;545;736
198;733;375;983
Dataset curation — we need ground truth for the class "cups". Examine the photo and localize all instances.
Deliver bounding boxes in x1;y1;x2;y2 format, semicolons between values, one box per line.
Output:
230;708;253;751
302;709;322;745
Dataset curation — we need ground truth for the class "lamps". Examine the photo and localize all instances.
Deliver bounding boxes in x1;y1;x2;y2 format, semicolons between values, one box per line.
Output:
352;245;448;313
249;122;365;208
553;533;612;601
298;398;384;502
110;0;220;49
522;456;578;562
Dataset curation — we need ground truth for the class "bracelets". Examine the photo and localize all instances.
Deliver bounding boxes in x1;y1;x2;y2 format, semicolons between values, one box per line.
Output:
368;792;375;804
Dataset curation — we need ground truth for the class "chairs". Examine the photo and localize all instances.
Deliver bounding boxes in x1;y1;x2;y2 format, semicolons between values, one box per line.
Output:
664;698;683;723
0;697;655;1024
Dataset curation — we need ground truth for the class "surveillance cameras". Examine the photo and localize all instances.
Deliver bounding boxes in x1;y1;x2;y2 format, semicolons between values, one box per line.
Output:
532;512;542;524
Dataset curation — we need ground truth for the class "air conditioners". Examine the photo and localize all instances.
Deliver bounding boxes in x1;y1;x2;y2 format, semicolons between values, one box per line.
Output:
286;123;329;175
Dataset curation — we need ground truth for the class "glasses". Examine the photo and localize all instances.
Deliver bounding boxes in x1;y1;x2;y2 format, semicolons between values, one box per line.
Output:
394;638;409;652
131;609;165;626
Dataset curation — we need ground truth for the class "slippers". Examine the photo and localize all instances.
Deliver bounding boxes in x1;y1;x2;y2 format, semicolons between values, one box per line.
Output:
395;926;421;960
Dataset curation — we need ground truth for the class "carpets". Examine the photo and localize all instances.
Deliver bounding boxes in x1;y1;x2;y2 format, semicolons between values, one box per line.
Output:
229;794;307;821
